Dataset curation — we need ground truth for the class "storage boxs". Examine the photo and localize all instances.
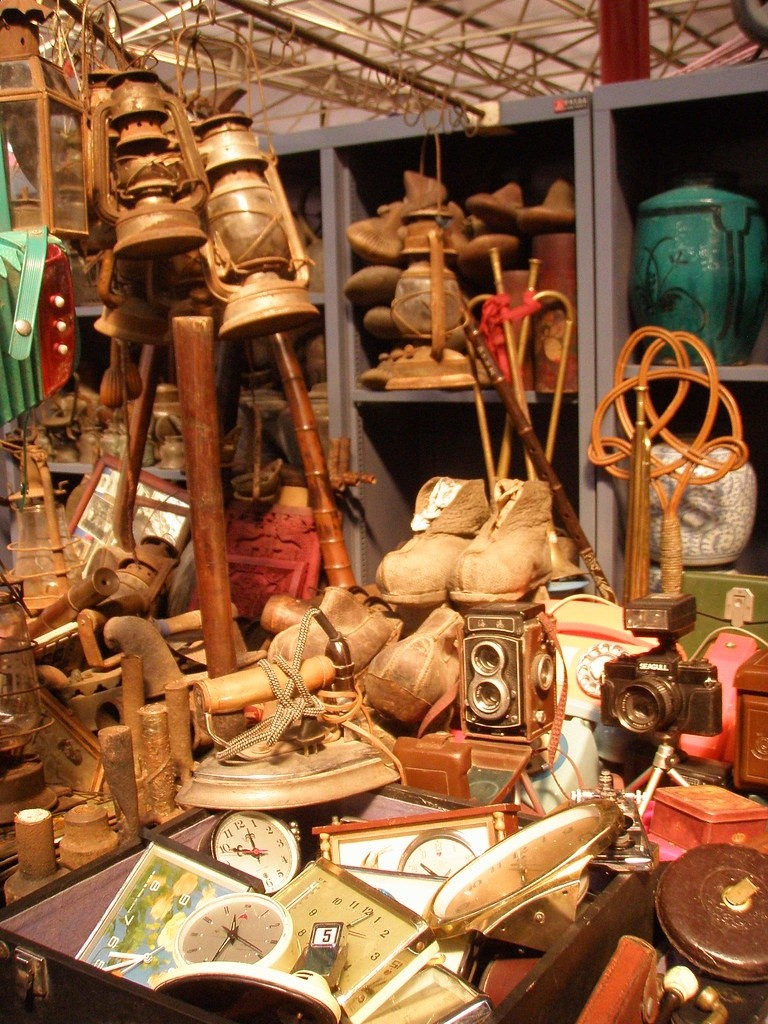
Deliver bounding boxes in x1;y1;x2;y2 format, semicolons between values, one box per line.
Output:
649;785;768;851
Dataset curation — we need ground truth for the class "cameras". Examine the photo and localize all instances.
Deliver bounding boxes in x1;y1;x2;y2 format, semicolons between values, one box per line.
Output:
600;593;723;738
461;602;559;742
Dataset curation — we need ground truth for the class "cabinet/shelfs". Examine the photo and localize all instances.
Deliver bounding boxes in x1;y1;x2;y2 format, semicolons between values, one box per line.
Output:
0;60;768;604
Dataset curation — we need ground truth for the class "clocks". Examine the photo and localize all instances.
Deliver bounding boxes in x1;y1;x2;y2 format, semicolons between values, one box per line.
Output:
265;857;434;1019
174;893;301;975
424;799;624;940
199;810;301;897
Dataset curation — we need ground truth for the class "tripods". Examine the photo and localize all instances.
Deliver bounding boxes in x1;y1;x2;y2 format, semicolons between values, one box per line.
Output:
625;737;691;819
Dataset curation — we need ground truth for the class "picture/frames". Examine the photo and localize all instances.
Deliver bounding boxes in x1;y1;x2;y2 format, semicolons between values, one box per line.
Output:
68;456;192;554
75;827;265;990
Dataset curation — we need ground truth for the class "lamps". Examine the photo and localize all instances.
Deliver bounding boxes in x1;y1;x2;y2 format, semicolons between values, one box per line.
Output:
6;448;82;611
94;250;188;346
93;68;210;262
0;568;58;829
383;127;479;391
194;112;320;341
